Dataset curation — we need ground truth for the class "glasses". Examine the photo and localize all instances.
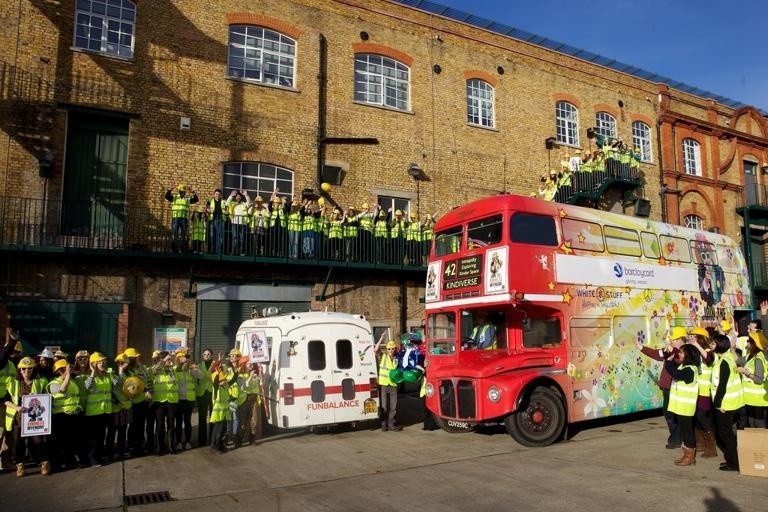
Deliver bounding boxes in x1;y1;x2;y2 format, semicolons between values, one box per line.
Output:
387;347;396;350
699;252;712;261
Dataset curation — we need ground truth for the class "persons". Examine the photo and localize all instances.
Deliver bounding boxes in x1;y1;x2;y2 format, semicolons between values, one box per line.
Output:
633;296;768;472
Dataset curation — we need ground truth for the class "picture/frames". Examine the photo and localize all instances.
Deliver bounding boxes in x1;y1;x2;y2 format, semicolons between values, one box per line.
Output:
246;331;270;364
20;393;52;438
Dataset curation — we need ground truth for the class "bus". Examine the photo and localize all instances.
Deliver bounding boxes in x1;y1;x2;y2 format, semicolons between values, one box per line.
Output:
424;190;756;454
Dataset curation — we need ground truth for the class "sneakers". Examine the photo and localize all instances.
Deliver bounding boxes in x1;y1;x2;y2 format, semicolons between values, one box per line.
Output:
665;440;682;449
45;433;256;468
422;425;437;432
40;461;50;476
15;462;24;478
171;246;429;268
719;462;740;473
380;425;404;432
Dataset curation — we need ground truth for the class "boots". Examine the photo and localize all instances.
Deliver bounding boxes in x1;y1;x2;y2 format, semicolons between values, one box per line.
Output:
696;429;718;459
674;446;696;467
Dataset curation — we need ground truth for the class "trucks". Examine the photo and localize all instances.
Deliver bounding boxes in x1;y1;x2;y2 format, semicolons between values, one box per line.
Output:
230;305;384;433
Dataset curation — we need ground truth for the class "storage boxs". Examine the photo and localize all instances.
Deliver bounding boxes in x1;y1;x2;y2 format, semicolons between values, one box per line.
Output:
736;427;768;478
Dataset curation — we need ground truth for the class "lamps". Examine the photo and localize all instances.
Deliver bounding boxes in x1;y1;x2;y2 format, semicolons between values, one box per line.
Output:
407;163;423;177
545;136;557;149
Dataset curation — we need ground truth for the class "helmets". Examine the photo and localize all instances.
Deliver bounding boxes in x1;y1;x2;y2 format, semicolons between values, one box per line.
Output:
550;169;557;176
561;137;644;169
747;330;768;351
668;326;688;340
385;339;398;348
687;326;710;339
177;181;434;221
13;342;255;385
545;180;552;185
530;192;538;198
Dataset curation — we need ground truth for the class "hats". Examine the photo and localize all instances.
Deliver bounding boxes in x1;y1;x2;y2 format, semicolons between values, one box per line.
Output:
121;375;146;399
